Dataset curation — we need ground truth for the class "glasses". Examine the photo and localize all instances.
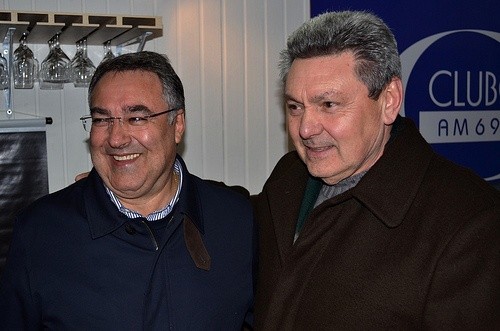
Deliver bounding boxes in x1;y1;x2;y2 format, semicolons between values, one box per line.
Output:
80;108;178;133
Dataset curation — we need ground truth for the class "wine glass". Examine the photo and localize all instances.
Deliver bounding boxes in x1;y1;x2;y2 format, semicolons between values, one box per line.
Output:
0;31;116;90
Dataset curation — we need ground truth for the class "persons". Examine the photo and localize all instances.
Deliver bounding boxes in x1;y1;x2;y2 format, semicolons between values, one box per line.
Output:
76;12;500;331
5;53;257;331
